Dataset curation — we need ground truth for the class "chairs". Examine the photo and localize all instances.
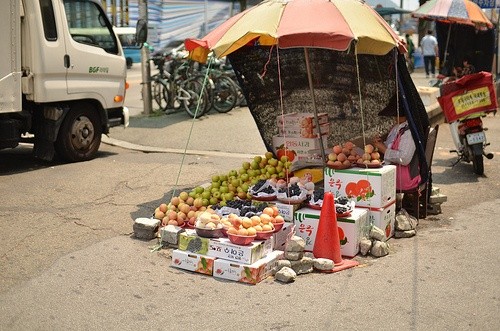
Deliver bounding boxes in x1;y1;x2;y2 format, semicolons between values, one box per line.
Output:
395;125;443;220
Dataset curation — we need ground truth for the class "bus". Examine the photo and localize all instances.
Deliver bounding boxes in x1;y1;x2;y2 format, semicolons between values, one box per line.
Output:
68;25;149;69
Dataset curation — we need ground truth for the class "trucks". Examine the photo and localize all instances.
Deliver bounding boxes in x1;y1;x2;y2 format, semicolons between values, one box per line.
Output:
0;0;148;163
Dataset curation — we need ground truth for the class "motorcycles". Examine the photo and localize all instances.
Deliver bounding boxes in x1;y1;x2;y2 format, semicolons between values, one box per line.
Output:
449;106;494;175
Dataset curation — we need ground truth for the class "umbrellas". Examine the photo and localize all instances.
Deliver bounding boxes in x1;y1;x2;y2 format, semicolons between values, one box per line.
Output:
185;1;409;166
411;0;493;61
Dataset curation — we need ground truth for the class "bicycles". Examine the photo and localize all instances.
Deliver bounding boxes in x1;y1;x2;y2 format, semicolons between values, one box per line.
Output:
141;49;295;118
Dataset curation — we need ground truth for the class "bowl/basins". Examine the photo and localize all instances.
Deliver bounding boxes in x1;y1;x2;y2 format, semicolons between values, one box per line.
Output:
151;212;285;247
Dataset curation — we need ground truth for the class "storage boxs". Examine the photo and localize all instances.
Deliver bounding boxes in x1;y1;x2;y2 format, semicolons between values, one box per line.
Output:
175;112;399;284
437;72;497;123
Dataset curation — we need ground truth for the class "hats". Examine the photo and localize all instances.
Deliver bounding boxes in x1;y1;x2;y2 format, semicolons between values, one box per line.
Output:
378;93;408;117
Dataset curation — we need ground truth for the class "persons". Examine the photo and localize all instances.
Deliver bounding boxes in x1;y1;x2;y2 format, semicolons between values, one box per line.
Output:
376;94;422;193
405;34;415;73
421;30;438;78
454;60;477;76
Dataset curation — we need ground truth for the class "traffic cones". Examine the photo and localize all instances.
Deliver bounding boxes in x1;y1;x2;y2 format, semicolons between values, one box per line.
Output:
312;192;359;273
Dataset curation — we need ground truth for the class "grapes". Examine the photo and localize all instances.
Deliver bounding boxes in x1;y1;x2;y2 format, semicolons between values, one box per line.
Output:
251;179;301;198
211;199;268;217
305;190;350;214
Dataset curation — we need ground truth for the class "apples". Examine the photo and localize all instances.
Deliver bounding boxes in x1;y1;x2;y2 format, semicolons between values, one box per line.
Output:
189;152;292;205
250;206;283;231
220;213;255;236
196;208;220;229
154;192;212;226
327;142;380;165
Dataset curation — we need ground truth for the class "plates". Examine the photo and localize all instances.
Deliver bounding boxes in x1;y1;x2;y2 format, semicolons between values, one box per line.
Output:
324;159;385;169
250;194;353;216
